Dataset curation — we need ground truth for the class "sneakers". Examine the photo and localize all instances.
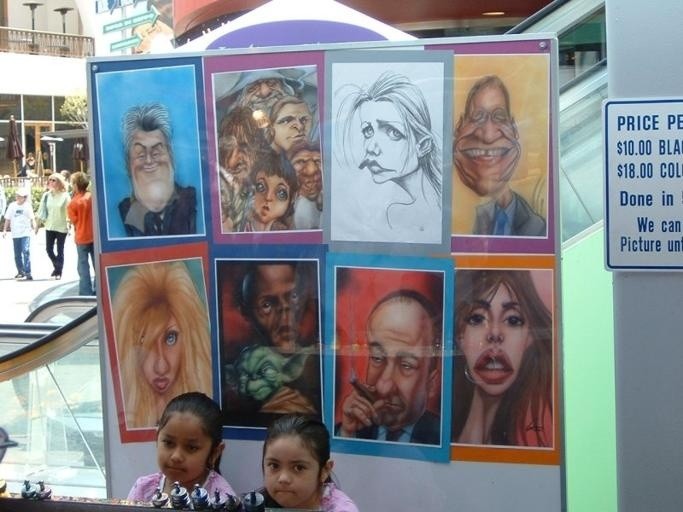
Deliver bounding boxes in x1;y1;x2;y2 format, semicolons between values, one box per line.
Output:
15;273;24;278
26;274;32;280
51;270;61;280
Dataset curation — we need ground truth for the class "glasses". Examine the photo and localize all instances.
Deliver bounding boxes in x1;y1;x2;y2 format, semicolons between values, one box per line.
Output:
49;179;58;182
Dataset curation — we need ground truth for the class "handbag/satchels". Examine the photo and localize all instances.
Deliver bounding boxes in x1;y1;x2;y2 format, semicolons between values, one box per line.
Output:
39;193;49;220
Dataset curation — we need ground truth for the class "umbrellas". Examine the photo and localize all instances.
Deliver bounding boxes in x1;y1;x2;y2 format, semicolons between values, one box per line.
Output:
73;137;90;172
6;114;23;177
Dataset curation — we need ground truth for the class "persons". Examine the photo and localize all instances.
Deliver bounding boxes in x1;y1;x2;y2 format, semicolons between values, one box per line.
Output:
25;152;35;177
3;169;97;296
255;413;359;511
128;391;240;508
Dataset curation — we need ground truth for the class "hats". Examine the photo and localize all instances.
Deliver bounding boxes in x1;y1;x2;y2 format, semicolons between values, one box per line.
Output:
16;188;29;197
